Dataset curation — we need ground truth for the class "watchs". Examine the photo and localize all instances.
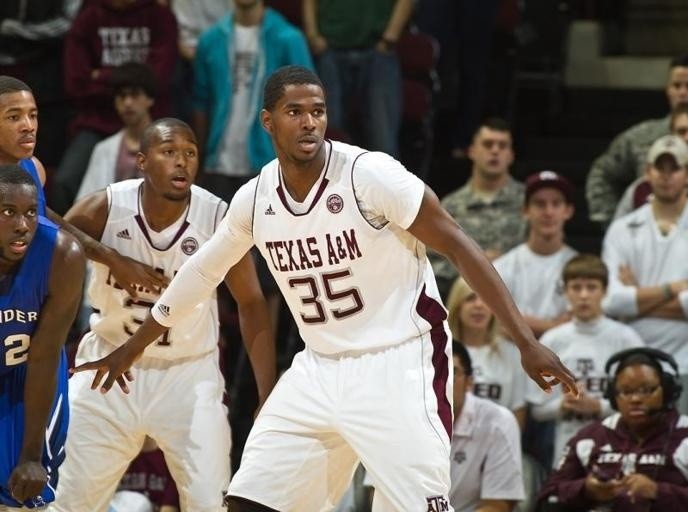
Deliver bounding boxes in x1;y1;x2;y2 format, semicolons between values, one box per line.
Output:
382;36;399;47
662;281;670;294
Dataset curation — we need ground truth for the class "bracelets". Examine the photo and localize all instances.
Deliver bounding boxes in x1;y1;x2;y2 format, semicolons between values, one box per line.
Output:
308;34;323;39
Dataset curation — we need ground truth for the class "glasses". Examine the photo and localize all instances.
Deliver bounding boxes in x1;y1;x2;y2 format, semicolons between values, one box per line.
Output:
614;382;663;398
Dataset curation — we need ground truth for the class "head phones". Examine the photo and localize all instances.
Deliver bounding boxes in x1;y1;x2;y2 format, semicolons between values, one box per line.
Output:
599;348;683;411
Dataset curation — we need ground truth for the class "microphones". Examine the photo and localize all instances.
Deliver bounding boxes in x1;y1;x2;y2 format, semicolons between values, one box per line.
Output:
645;407;670;416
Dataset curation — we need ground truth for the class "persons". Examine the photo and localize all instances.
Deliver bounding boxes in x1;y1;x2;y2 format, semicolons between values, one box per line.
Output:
529;347;688;512
607;101;688;222
49;119;274;512
425;116;528;300
492;171;579;338
364;340;524;512
189;1;319;204
55;1;178;212
0;75;171;303
75;61;161;210
602;136;688;411
585;59;688;229
303;0;411;164
525;255;646;469
448;274;527;434
1;167;86;509
2;0;80;139
69;66;579;512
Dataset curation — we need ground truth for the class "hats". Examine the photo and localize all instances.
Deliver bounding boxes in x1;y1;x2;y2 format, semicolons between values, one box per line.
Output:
524;168;574;202
645;135;684;165
